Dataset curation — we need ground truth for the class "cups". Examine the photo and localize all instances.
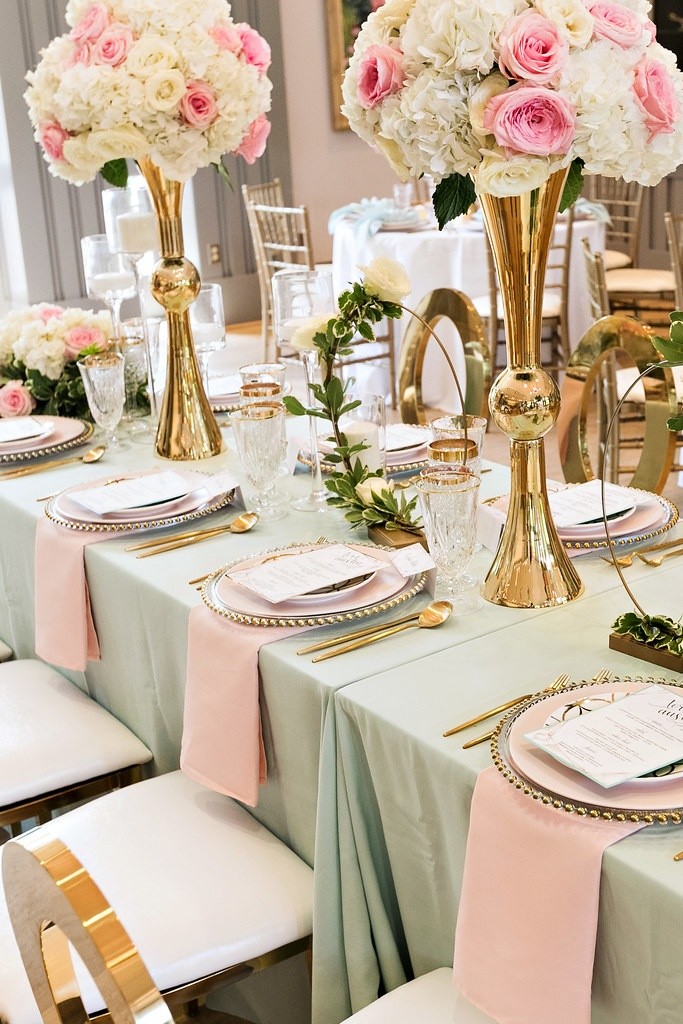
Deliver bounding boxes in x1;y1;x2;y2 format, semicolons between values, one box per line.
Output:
394;182;411;210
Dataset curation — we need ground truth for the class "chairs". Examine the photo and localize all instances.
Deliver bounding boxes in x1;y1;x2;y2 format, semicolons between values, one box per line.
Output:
0;167;683;1024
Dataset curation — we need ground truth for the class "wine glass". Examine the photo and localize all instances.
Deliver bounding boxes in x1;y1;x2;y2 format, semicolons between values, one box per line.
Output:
65;182;389;517
417;414;487;613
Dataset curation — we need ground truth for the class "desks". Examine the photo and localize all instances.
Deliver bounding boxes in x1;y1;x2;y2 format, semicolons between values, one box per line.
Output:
330;196;608;414
331;566;683;1024
0;413;683;1024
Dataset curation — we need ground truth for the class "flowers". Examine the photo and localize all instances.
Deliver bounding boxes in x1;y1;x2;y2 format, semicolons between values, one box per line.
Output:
280;250;424;535
0;300;152;425
24;0;274;193
338;0;683;231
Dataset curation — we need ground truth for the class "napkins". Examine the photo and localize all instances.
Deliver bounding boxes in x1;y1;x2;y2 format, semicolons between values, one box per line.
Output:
34;516;133;672
453;765;650;1024
179;604;324;805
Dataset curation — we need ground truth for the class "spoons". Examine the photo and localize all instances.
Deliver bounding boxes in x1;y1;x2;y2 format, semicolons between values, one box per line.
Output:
137;512;259;559
312;600;454;663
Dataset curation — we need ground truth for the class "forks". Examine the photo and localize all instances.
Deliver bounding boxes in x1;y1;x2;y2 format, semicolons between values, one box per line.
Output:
598;537;683;568
443;668;613;749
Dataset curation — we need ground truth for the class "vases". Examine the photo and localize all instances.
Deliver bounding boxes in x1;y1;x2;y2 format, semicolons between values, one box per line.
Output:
468;165;586;610
135;155;226;462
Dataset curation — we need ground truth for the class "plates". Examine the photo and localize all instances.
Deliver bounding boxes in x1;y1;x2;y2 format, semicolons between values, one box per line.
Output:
296;421;430;477
201;540;431;626
488;484;679;546
1;414;94;465
488;675;683;831
44;467;234;533
